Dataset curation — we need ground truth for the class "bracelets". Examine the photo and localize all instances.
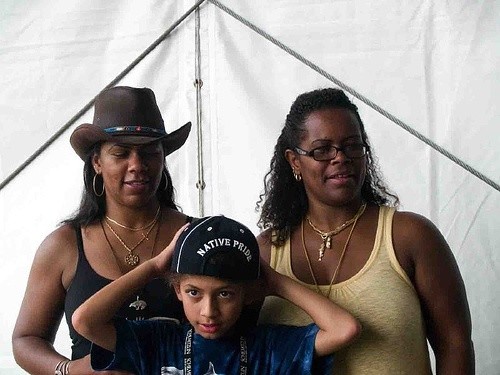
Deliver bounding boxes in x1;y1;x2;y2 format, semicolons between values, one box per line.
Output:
54;359;71;375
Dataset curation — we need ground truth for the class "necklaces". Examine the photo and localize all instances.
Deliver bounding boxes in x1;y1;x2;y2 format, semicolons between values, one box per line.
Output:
306;203;368;262
301;202;365;299
99;204;162;321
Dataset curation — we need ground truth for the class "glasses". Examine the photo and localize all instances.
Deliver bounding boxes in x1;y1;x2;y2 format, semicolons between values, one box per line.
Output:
291;139;370;161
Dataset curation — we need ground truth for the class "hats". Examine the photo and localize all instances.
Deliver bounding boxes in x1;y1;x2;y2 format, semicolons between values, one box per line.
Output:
71;87;191;162
172;216;260;279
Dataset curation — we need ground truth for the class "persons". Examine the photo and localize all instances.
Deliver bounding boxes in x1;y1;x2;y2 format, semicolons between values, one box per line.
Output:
72;217;360;375
13;86;200;375
253;87;477;375
